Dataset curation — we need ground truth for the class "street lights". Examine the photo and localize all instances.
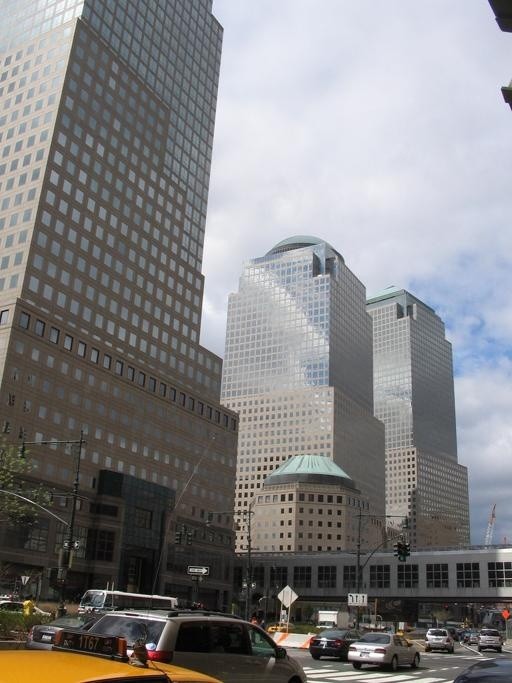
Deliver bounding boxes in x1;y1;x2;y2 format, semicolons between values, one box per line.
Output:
205;507;255;622
398;541;405;561
404;542;410;556
354;506;408;628
393;542;399;556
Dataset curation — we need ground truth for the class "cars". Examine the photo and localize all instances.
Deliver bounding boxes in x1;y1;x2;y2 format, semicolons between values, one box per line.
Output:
26;611;106;648
310;627;363;662
0;627;224;682
347;630;421;671
0;600;51;617
454;654;512;682
445;625;504;652
267;621;298;633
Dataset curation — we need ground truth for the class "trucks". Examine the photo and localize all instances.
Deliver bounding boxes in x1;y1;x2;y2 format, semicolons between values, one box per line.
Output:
316;609;351;629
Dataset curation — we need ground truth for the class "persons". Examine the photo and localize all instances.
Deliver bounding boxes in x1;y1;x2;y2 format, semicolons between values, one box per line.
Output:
259;620;265;641
249;616;258;644
22;596;35;622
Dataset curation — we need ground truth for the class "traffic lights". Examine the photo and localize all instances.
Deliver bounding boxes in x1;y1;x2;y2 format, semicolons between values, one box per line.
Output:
444;602;449;610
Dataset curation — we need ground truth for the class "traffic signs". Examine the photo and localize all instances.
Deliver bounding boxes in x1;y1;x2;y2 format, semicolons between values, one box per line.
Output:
346;592;368;607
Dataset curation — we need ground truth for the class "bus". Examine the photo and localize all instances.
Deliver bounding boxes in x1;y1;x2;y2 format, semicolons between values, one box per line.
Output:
78;589;179;619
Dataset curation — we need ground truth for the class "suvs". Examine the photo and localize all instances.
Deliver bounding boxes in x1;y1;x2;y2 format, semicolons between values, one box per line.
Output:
85;605;308;681
424;626;455;653
